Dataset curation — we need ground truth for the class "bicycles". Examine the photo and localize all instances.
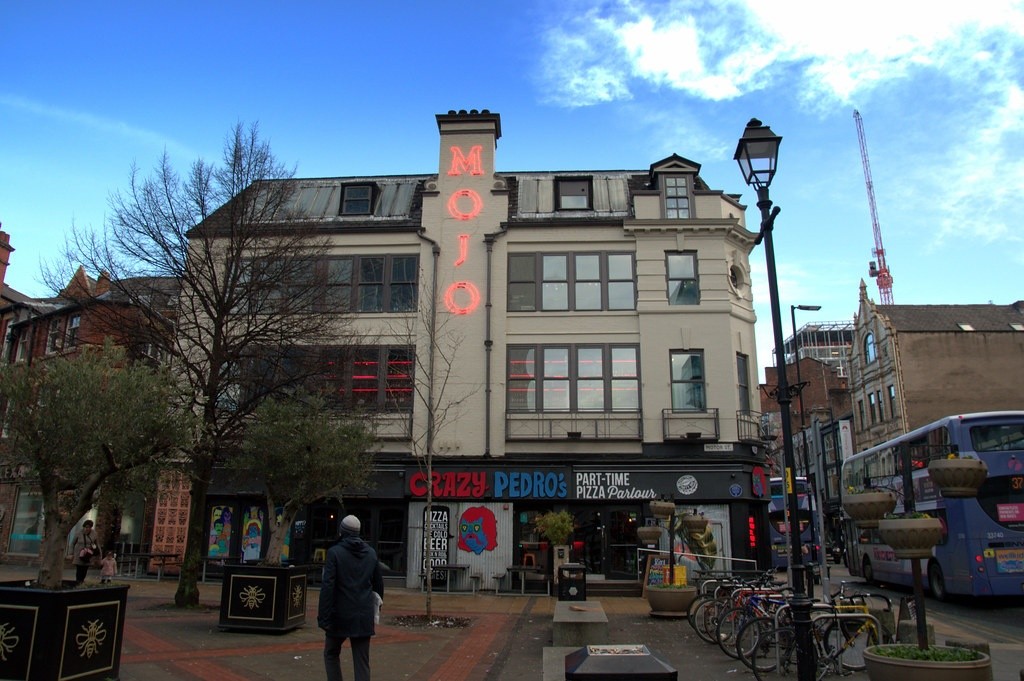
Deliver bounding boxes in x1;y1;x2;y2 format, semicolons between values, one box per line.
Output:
686;574;894;681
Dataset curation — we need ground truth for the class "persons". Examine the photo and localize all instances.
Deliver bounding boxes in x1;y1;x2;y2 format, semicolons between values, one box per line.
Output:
27;522;39;535
98;551;117;584
317;514;384;681
70;520;102;581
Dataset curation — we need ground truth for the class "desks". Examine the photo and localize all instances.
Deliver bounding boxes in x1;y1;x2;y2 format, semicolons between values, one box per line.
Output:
507;565;541;594
693;569;765;579
432;564;470;593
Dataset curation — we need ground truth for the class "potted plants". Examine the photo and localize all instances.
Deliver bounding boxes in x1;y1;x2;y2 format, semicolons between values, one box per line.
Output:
217;386;382;635
0;338;194;681
650;501;675;518
637;526;661;543
646;584;698;617
864;643;994;681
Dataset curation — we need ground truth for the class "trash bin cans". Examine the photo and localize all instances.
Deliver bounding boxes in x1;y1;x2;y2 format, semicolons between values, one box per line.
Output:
557;564;587;601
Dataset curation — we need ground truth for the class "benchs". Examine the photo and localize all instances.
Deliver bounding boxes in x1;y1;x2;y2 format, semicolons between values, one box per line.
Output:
470;572;481;596
418;572;433;591
493;573;506;593
544;569;553;595
692;575;732;582
553;600;608;648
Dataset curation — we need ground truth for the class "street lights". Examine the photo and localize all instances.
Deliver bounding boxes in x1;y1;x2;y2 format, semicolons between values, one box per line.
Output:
791;305;821;598
732;118;819;680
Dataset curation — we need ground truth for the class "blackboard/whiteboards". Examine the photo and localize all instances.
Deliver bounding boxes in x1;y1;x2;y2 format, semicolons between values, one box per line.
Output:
643;554;676;591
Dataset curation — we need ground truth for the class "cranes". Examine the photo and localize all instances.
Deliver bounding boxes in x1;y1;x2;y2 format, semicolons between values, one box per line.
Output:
853;110;894;305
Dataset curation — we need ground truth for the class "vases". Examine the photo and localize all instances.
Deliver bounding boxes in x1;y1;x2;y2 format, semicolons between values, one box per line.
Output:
878;518;943;558
928;458;987;497
842;492;897;528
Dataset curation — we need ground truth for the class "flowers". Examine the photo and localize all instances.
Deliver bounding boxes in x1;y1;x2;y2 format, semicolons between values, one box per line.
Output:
847;486;881;494
947;454;974;459
885;511;930;520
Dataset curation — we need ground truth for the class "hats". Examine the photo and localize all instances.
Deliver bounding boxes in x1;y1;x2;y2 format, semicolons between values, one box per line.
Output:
340;515;360;535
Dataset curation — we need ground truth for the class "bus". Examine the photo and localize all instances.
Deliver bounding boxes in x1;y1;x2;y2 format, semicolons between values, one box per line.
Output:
839;411;1024;603
768;477;819;572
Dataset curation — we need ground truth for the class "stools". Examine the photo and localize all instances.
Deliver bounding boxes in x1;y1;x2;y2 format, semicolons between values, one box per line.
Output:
524;553;536;567
314;548;327;563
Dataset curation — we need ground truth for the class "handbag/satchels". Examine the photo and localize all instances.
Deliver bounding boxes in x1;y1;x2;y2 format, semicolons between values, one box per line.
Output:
79;548;93;562
